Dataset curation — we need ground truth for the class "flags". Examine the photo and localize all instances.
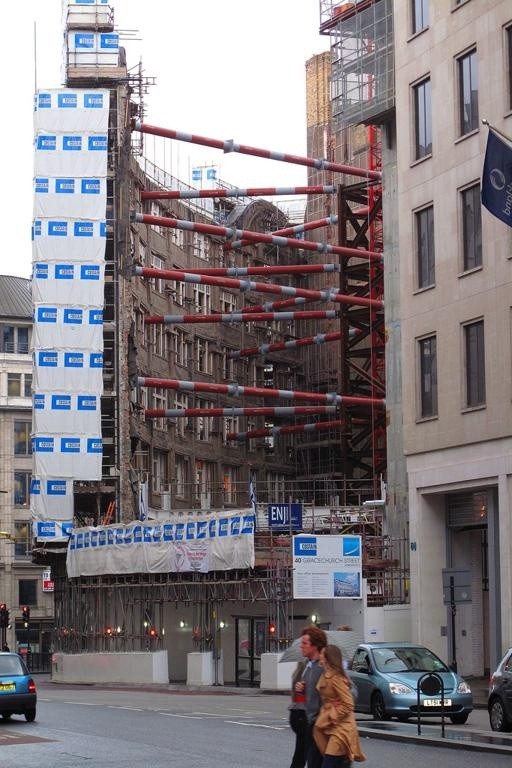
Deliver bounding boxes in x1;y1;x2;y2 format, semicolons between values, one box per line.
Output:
139;485;147;522
246;476;257;510
479;129;511;226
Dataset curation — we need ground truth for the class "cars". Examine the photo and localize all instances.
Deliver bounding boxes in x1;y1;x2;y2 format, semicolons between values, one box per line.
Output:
342;641;474;725
485;646;512;732
0;650;37;722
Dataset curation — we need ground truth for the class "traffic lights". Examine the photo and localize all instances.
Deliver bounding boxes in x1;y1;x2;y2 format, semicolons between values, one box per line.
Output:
22;605;31;622
0;604;6;628
6;611;10;627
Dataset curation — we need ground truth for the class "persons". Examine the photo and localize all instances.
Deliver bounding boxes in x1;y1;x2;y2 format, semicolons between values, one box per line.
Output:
312;645;367;768
288;662;310;768
2;641;10;651
296;625;360;768
